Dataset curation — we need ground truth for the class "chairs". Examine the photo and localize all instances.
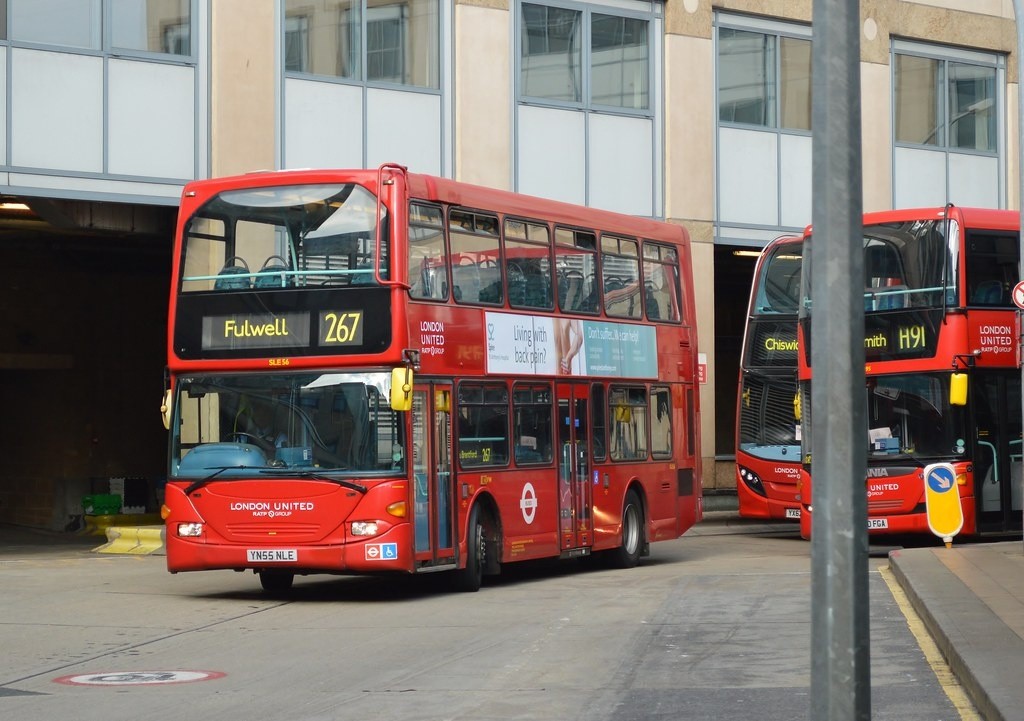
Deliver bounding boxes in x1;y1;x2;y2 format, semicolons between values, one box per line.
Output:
215;256;250;290
351;250;387;285
253;255;292;289
514;439;546;464
419;252;668;324
973;280;1002;304
576;436;604;458
457;427;496;467
863;285;912;313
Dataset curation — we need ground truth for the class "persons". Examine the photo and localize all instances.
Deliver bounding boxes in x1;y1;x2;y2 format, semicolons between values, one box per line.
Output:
234;400;290;458
553;317;584;375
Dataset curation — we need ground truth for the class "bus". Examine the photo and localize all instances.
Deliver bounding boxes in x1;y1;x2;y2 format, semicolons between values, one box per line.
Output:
160;163;704;593
736;237;902;520
797;204;1024;539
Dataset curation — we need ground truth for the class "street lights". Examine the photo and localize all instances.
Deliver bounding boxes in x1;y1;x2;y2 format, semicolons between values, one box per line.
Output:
922;98;994;145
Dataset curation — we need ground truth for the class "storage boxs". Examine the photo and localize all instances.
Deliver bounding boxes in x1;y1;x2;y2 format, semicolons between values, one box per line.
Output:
109;476;147;515
80;493;122;515
